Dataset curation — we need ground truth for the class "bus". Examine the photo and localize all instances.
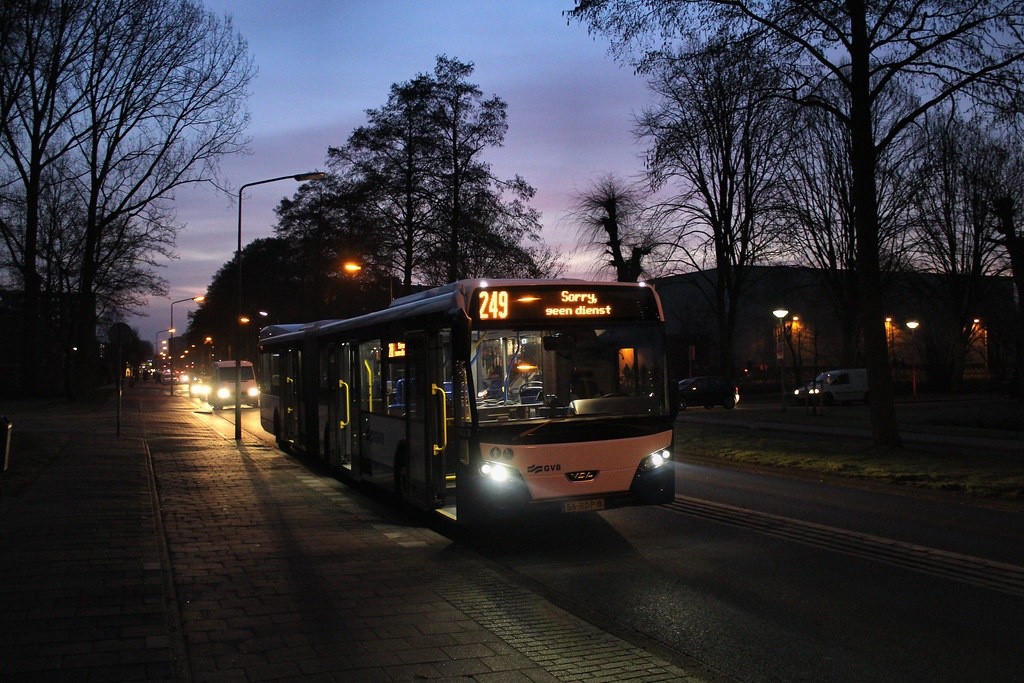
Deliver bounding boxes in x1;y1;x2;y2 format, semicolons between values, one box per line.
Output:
259;278;674;540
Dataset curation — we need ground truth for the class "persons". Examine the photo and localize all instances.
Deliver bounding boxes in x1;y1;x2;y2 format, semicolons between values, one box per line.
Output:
623;364;648;385
485;366;504;397
143;371;148;380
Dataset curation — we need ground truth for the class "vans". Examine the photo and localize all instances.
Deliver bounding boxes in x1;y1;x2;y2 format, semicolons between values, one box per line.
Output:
793;369;869;407
210;361;259;410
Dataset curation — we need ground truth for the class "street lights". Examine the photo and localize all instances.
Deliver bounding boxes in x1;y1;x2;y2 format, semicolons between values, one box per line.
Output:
234;169;328;444
169;295;204;397
906;321;922;399
156;327;176;376
180;315;263;373
260;311;280;324
773;306;788;393
348;264;394;307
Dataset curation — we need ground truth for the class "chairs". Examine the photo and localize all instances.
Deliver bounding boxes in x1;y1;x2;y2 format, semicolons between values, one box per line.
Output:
383;377;544;411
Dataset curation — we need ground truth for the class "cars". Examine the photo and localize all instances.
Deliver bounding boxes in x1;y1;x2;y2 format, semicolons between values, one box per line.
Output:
155;369;197;384
678;377;740;410
